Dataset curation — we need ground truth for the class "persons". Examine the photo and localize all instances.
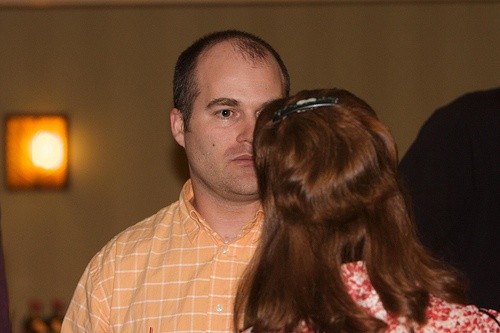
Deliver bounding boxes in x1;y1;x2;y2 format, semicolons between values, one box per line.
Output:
60;28;289;333
393;89;500;312
232;87;500;332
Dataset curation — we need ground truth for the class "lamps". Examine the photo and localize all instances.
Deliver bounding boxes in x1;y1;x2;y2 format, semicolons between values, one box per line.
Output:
4;111;71;192
